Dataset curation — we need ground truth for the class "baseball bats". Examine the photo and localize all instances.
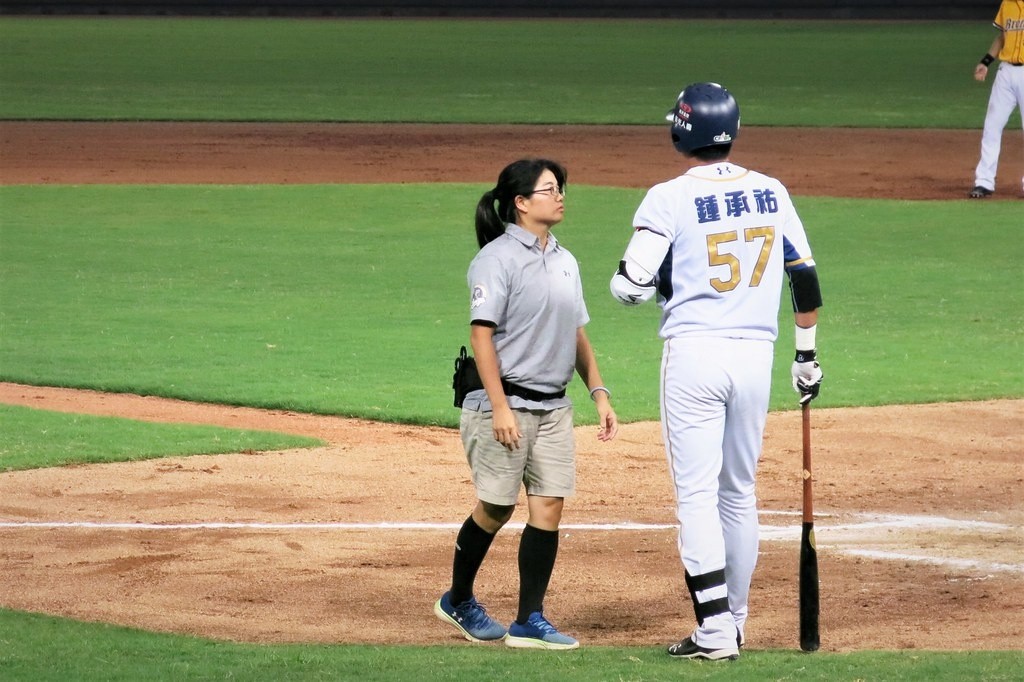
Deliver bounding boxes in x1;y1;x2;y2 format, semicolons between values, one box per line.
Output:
797;373;821;652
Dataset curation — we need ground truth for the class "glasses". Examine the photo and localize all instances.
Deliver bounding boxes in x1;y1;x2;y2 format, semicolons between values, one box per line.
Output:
529;185;567;196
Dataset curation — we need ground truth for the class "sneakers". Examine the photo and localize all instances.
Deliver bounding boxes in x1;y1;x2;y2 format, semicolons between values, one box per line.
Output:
433;591;508;643
667;637;740;660
506;610;579;650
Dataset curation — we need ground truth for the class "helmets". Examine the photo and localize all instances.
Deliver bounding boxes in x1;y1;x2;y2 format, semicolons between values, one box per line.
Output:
666;82;741;150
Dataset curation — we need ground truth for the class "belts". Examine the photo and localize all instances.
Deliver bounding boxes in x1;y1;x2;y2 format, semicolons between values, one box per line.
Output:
1011;63;1022;66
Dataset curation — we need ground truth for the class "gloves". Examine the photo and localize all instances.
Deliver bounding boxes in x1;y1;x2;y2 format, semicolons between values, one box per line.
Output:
790;348;822;405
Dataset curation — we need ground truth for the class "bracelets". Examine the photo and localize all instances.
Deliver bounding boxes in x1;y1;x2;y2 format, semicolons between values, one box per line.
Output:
981;53;995;67
589;387;612;403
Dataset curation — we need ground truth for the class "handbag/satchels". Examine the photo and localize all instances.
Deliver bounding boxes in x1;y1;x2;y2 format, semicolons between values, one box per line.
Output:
452;354;565;408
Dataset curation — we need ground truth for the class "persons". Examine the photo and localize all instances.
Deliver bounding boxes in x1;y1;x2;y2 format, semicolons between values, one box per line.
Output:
433;158;618;650
609;81;824;662
968;0;1024;199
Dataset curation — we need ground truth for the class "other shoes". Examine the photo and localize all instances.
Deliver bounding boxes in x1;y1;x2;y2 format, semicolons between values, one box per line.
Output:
970;186;991;197
